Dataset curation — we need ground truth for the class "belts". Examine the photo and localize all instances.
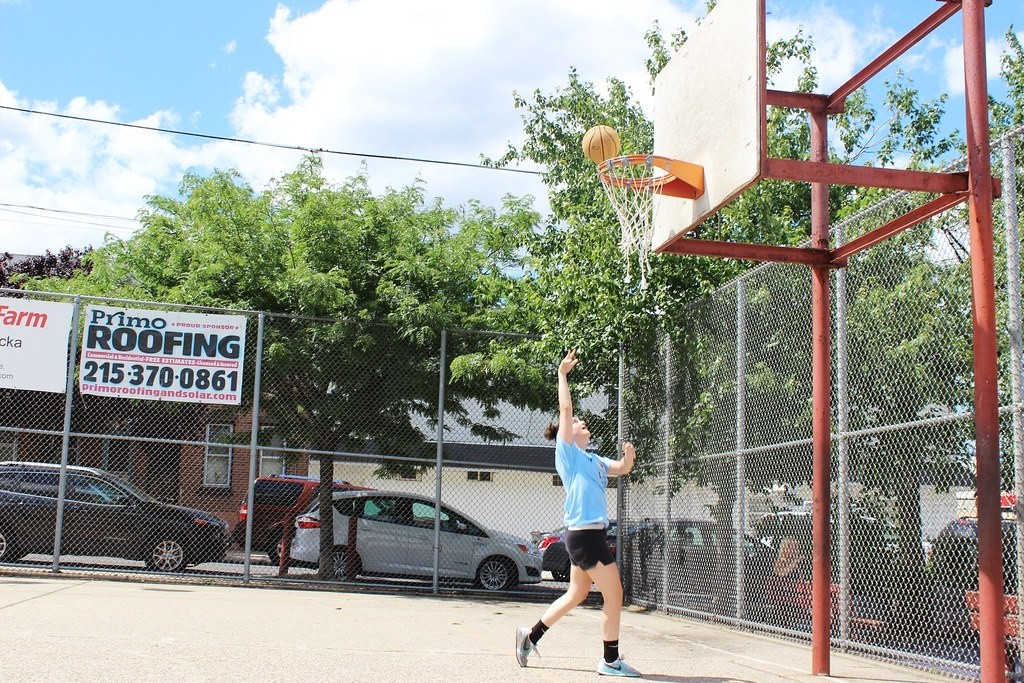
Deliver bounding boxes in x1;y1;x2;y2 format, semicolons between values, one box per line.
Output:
568;524;604;530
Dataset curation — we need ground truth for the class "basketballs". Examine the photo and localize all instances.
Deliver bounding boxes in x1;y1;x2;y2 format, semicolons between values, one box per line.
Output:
582;125;620;164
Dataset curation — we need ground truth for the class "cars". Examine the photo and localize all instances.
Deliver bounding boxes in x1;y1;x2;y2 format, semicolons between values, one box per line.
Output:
289;489;541;591
533;500;1017;644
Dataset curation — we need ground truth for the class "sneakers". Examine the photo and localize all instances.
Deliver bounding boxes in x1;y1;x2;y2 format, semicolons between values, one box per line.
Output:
515;626;541;668
597;654;640;676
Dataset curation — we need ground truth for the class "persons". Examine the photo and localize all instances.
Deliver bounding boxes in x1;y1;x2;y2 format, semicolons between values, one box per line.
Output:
516;348;641;676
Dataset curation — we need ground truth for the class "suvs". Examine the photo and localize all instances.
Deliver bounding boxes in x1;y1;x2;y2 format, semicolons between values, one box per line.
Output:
0;460;230;571
232;473;444;565
